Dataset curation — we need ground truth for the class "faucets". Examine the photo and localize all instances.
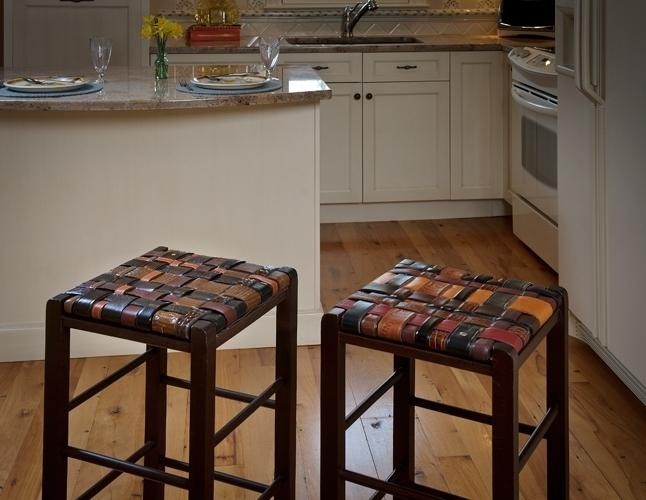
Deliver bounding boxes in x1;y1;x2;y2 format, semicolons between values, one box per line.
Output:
344;0;380;38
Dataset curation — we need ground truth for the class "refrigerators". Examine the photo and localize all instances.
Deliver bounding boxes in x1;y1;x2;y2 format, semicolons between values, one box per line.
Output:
558;0;646;403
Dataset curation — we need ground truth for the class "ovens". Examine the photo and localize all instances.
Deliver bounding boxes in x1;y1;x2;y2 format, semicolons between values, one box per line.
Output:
507;47;558;230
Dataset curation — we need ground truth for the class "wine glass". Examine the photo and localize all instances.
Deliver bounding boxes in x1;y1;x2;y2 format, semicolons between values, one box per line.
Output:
259;37;280;81
89;37;111;84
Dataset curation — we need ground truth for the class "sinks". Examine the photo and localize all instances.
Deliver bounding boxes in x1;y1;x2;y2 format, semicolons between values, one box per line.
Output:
285;36;424;45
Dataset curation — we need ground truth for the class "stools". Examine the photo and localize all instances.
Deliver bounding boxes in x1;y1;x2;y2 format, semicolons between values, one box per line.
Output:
320;258;568;499
41;245;297;500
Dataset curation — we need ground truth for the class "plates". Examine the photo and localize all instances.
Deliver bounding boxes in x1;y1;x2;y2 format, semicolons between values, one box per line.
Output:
3;76;90;93
190;73;267;90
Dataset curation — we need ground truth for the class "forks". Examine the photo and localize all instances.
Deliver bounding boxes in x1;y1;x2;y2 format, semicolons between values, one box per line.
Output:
178;80;195;92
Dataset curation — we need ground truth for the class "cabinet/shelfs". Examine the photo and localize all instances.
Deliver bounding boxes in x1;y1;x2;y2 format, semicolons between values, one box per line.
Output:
151;53;513;226
